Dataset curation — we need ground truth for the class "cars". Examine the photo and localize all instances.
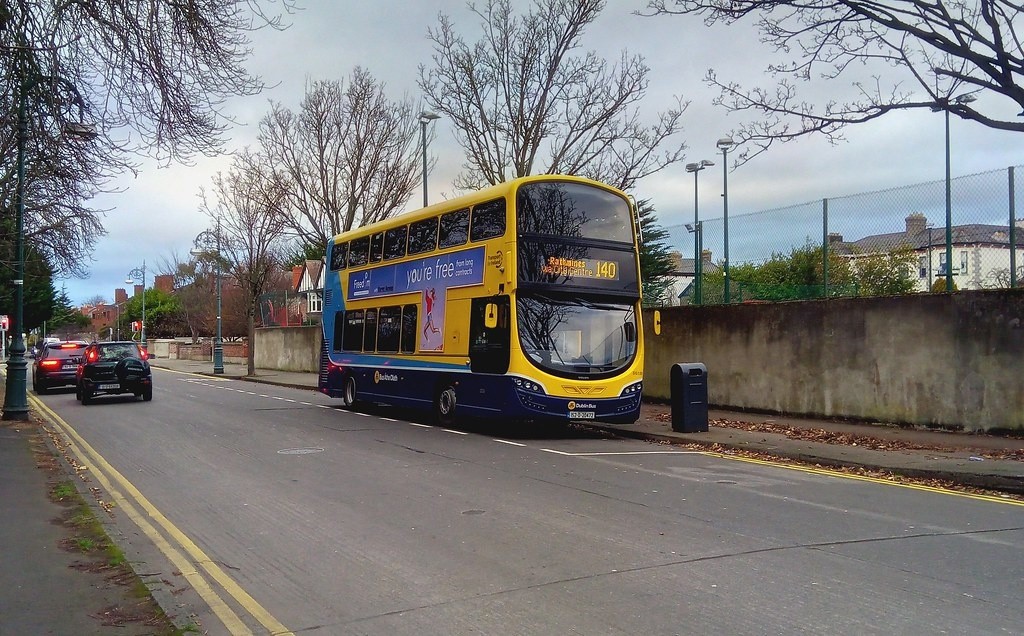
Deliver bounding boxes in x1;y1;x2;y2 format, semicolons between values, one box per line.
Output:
72;341;153;406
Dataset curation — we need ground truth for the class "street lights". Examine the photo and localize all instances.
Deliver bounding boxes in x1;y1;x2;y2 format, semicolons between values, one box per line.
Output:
2;75;98;422
931;95;978;291
189;230;224;374
416;110;442;209
114;305;120;341
685;159;714;305
715;137;733;304
125;269;148;361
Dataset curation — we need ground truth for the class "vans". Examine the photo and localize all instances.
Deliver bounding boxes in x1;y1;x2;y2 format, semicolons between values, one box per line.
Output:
34;337;60;360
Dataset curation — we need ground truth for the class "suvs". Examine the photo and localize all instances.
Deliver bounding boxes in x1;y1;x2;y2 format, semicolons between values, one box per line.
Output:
30;341;91;394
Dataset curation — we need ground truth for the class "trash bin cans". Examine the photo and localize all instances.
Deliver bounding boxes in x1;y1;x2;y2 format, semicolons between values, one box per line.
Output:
671;362;709;431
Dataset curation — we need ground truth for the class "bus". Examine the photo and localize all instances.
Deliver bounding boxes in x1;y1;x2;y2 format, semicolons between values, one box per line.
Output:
318;176;663;431
